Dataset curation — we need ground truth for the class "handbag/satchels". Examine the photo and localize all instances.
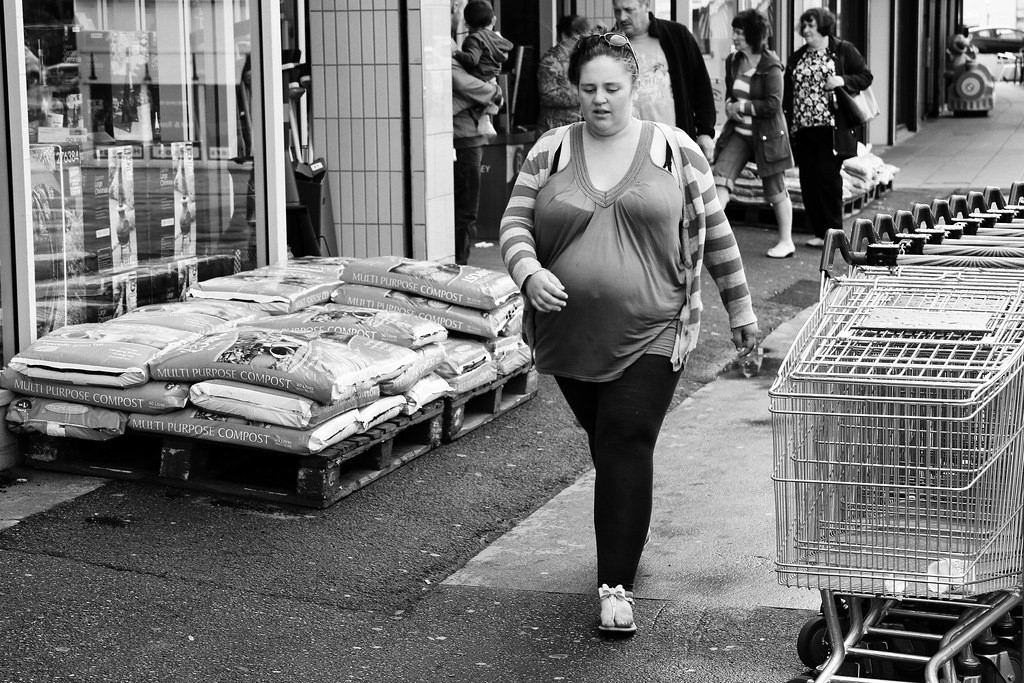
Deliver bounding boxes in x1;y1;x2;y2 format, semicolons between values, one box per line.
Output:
850;85;879;123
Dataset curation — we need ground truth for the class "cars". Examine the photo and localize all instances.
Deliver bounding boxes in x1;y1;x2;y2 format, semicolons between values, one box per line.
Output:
966;24;1024;54
24;45;43;90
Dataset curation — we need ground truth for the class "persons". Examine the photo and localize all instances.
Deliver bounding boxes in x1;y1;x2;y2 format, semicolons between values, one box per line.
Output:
498;32;759;635
537;15;592;130
451;0;516;266
604;0;716;162
711;10;796;259
782;7;873;249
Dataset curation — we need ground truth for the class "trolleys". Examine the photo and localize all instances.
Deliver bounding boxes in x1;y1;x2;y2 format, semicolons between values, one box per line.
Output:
769;179;1024;683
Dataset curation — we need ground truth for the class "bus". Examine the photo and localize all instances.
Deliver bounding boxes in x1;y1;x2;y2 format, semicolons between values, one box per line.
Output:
45;63;84;113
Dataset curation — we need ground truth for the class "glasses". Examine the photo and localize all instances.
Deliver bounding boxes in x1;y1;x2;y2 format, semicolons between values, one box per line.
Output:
577;33;639;74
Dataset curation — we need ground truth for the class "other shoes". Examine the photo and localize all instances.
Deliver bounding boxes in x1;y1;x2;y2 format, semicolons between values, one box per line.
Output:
477;115;497;135
767;242;795;258
806;238;824;247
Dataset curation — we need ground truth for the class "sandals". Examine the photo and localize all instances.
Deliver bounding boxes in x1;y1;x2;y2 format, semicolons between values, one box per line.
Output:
598;584;638;631
